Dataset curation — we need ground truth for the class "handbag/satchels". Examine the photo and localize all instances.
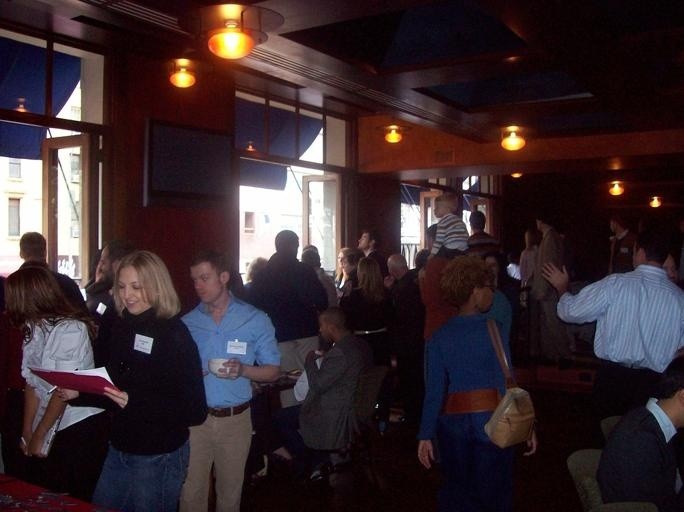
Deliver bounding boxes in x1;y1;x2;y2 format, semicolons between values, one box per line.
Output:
484;387;537;448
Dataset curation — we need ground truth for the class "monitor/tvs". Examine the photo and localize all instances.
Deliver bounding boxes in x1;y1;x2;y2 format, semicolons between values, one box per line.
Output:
142;118;235;208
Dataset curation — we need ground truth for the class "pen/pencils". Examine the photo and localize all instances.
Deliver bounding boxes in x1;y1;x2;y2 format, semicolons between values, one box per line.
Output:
21;436;27;447
46;368;78;395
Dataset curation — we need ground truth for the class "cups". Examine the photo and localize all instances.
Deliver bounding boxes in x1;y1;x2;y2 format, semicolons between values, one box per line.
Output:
209;358;230;377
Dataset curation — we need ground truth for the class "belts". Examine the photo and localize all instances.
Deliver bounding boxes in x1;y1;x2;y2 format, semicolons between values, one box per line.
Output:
207;402;250;417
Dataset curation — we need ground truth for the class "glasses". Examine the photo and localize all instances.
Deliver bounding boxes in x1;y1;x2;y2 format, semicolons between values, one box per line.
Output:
472;282;495;291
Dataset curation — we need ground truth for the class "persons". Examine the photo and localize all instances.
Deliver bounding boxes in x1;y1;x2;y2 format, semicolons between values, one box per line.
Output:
1;192;683;511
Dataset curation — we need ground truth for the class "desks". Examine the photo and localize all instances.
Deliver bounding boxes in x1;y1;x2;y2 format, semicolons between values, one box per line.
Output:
0;472;125;512
249;368;309;419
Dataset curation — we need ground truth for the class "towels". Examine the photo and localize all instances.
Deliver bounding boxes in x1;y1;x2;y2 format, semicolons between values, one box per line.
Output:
293;349;324;401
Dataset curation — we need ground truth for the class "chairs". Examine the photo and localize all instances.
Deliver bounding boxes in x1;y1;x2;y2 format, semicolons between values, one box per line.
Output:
301;377;383;496
600;415;623;438
567;449;656;512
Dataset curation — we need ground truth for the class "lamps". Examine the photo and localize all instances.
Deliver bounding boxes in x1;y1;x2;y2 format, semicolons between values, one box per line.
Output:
170;68;197;88
384;129;402;143
609;183;624;196
649;198;661;207
501;131;525;151
206;20;254;59
